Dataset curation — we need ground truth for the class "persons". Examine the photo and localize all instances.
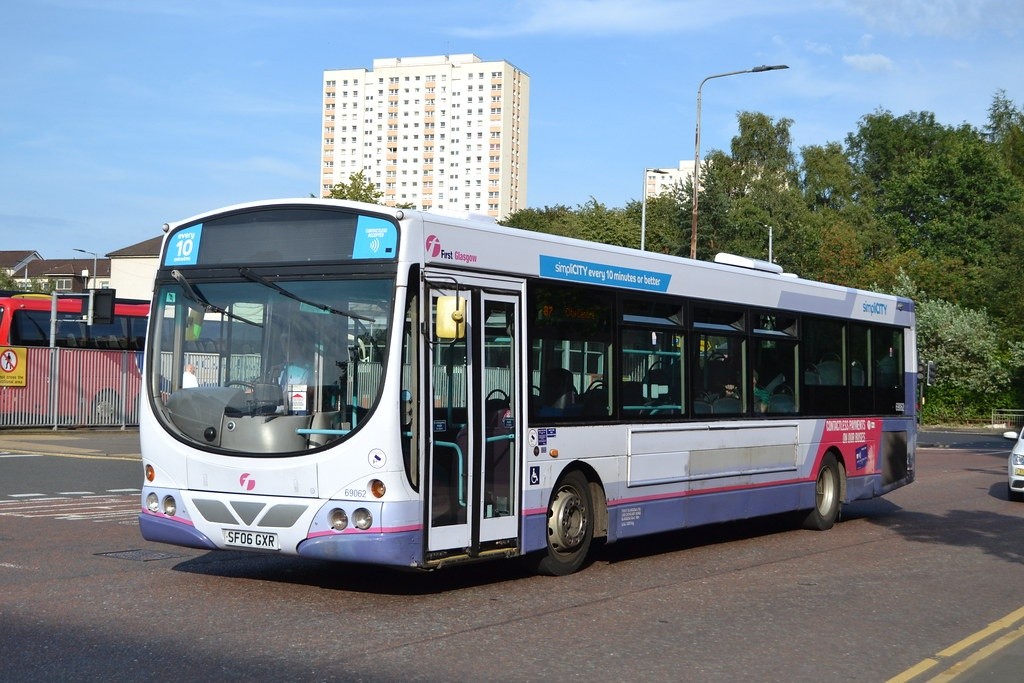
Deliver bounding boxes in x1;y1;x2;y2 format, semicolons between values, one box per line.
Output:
725;370;770;412
244;322;315;406
183;364;199;388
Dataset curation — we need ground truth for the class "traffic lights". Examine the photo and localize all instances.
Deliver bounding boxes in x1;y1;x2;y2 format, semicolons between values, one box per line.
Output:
927;360;935;386
918;362;925;383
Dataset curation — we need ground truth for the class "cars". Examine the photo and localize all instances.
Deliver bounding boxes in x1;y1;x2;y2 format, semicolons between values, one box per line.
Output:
1003;425;1024;499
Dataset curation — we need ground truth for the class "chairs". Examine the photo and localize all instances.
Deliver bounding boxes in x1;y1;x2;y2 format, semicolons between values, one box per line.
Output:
484;349;897;428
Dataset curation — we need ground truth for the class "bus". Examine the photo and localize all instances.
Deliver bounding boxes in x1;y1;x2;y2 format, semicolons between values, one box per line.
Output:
139;198;918;576
0;290;221;422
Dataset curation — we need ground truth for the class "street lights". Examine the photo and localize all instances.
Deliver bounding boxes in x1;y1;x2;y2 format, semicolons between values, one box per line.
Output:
641;168;670;250
73;249;96;288
690;65;789;260
756;222;772;264
18;260;27;289
